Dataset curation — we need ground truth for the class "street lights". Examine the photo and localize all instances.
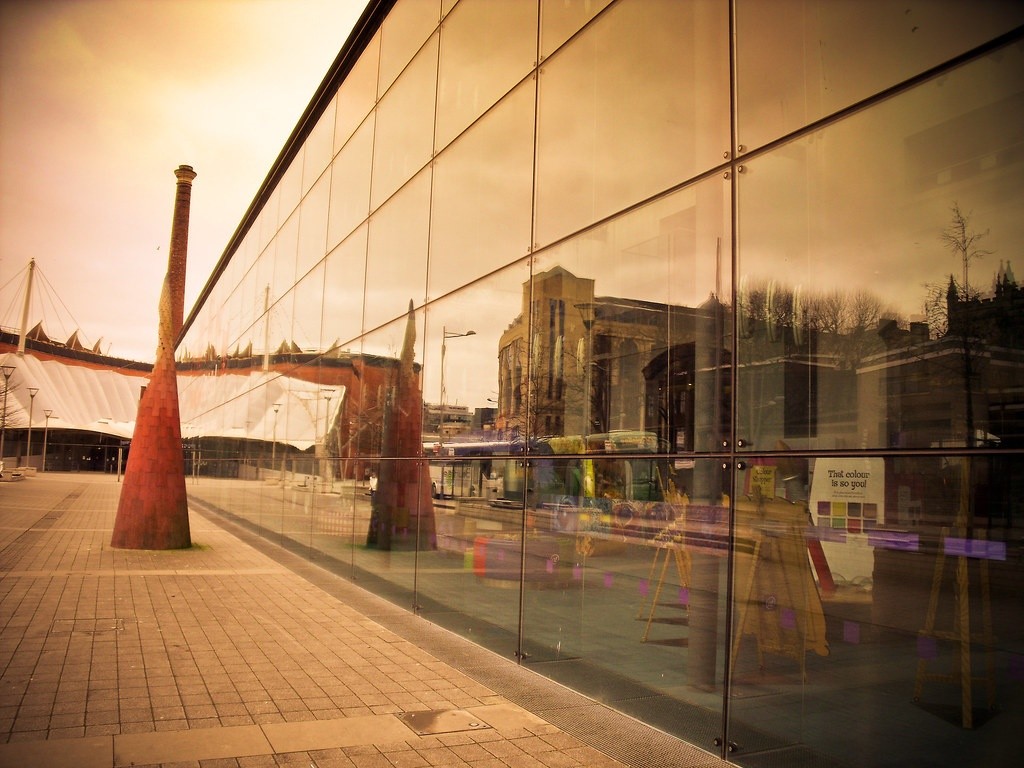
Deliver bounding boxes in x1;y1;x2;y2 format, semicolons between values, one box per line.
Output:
42;409;53;472
0;366;18;463
25;387;40;468
439;327;477;451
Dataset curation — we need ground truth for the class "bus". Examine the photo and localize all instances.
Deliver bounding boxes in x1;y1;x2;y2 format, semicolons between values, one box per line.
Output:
502;430;678;513
421;440;513;502
